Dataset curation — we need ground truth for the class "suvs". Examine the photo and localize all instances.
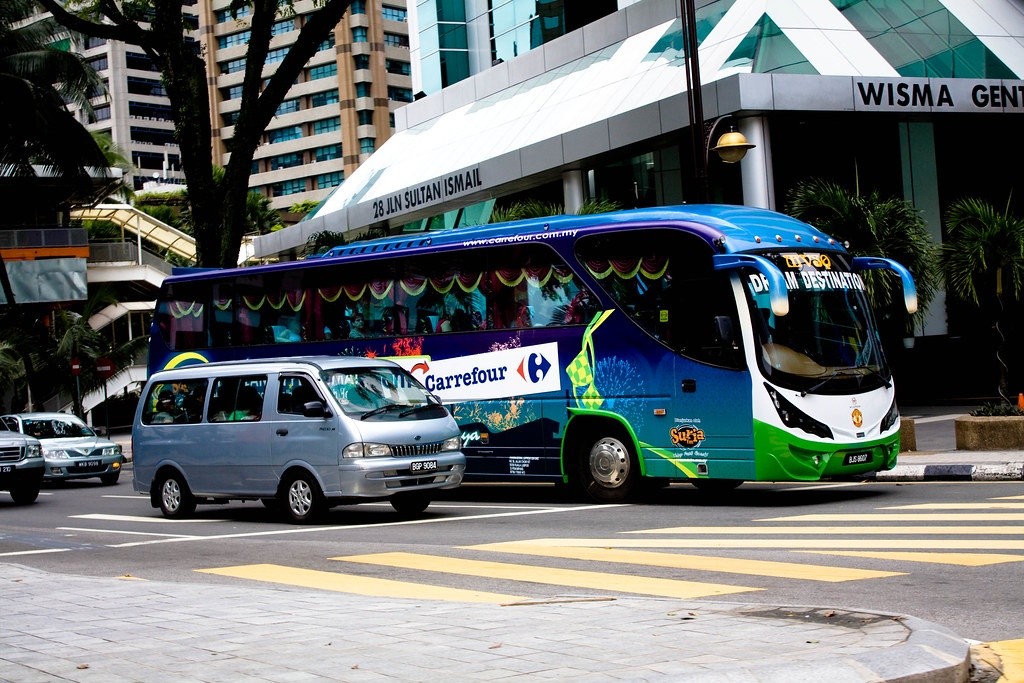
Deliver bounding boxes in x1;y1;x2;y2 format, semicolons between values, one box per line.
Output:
0;418;44;506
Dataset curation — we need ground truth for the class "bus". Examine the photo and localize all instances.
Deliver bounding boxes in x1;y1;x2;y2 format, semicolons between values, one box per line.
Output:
145;199;919;507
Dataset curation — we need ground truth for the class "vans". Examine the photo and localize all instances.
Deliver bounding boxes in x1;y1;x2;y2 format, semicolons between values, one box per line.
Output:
130;353;468;532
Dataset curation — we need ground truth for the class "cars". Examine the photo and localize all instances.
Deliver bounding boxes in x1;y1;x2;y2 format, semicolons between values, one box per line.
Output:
1;412;126;488
